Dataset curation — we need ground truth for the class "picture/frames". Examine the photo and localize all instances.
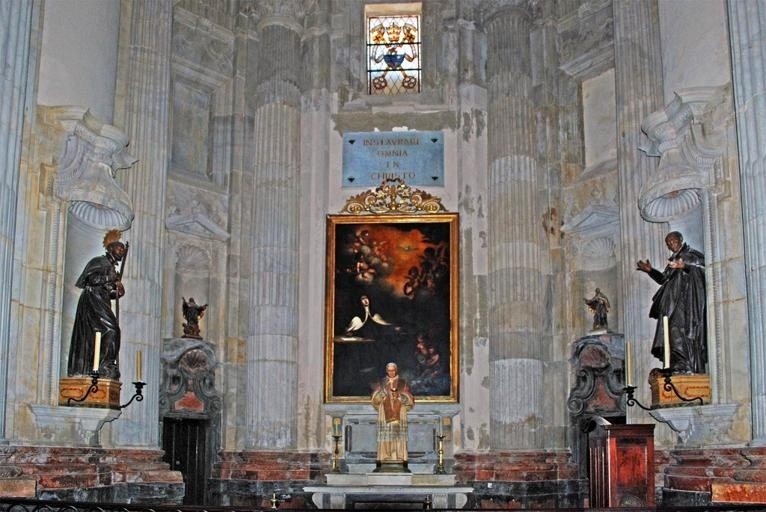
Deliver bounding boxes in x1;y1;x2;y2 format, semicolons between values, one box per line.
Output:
322;210;459;404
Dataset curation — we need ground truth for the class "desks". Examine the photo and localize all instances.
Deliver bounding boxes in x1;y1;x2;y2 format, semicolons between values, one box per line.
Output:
302;486;473;510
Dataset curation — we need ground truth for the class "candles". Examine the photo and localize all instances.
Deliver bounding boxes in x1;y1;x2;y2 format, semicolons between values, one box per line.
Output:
92;332;102;372
439;415;442;437
625;340;632;386
661;314;670;367
335;418;339;434
136;350;142;379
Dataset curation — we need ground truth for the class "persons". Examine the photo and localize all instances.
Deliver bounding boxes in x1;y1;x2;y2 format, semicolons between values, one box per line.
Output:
583;288;610;331
182;295;208;337
68;241;125;379
636;231;707;375
341;229;450;380
371;362;415;473
